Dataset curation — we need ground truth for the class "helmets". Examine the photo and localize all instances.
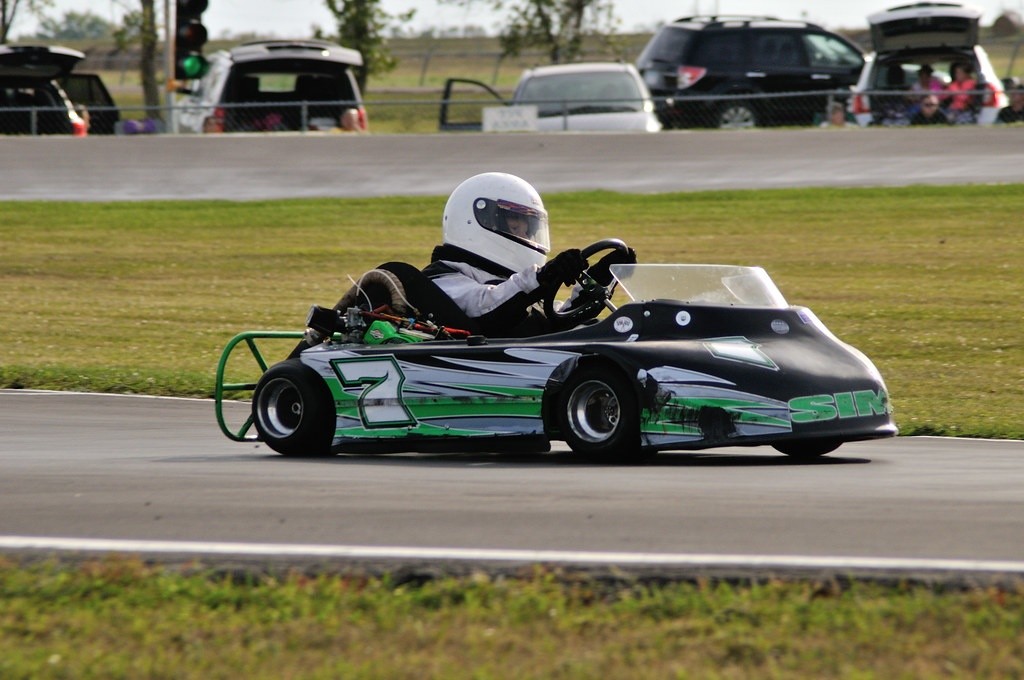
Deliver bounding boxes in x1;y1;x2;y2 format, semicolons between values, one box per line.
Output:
443;172;548;278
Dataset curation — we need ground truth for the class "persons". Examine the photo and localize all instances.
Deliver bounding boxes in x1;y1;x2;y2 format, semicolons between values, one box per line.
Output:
422;172;637;343
340;107;365;133
942;62;978;116
1003;79;1015;92
882;63;910;113
995;83;1024;123
912;64;947;102
68;106;90;135
817;101;857;129
908;94;952;126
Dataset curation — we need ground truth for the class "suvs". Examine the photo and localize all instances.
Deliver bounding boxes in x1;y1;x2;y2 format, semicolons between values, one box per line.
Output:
634;14;869;130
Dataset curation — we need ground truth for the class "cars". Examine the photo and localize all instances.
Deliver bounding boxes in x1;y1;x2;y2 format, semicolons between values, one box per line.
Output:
0;44;119;136
849;1;1008;125
176;38;367;133
437;62;662;132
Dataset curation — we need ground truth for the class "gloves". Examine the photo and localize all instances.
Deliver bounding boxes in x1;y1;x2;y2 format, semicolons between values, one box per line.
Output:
537;249;589;300
587;247;637;287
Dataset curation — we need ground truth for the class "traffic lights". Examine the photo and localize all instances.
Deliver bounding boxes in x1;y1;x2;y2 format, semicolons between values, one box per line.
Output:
176;0;207;93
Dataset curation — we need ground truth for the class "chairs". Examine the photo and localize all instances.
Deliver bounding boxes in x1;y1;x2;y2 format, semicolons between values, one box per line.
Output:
240;74;261;91
295;74;315;88
